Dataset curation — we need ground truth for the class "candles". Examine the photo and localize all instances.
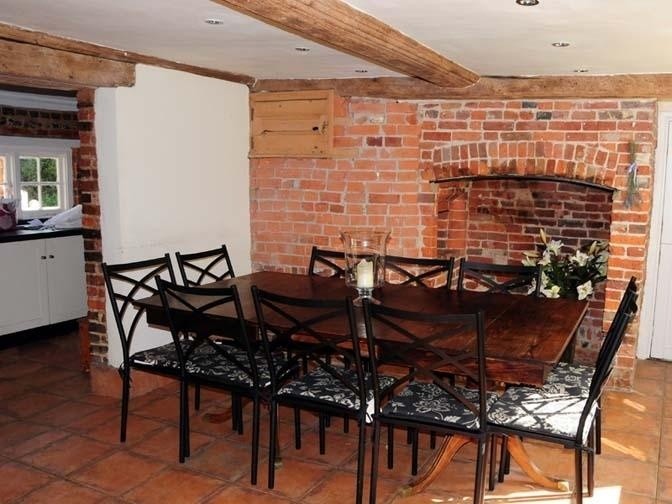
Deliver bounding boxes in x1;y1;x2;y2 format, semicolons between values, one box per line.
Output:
354;257;375;288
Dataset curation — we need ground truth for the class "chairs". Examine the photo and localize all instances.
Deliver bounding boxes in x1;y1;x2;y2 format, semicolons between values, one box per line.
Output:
478;276;637;504
383;254;453;292
307;245;378;285
249;283;395;504
363;298;506;504
155;274;304;486
101;252;240;458
459;261;542;300
173;245;243;434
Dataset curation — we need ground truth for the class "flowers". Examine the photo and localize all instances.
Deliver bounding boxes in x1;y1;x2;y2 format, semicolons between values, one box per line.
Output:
517;227;611;304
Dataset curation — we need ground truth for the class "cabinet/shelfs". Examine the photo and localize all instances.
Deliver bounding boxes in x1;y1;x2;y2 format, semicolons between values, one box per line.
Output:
1;235;88;338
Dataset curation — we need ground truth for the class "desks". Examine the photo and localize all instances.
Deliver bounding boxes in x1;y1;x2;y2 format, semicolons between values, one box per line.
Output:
131;272;589;497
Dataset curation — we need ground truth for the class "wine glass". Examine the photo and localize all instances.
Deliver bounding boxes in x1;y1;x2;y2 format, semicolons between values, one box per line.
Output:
340;229;391;308
